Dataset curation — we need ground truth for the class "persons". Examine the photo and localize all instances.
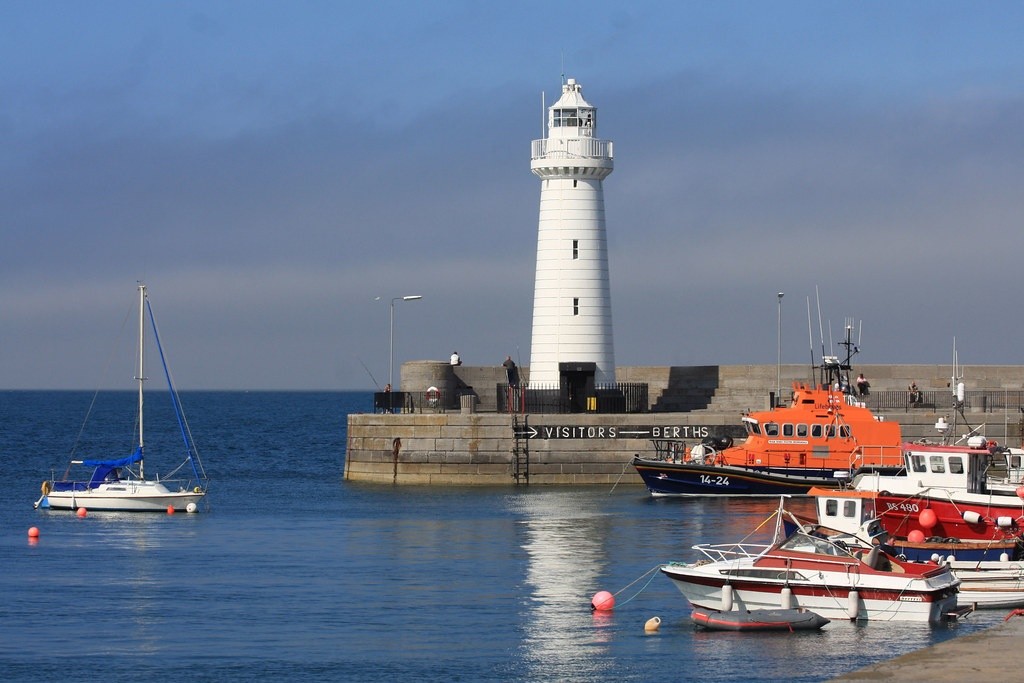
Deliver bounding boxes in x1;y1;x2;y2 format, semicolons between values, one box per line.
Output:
501;356;515;388
669;442;678;462
909;381;918;401
450;351;462;366
111;468;124;482
383;385;390;413
857;373;871;402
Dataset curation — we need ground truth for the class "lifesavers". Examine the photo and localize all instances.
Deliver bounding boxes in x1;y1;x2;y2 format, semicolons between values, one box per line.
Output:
41;481;50;495
425;386;440;402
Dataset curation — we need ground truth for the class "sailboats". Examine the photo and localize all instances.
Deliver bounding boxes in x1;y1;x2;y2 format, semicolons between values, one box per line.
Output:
31;277;211;513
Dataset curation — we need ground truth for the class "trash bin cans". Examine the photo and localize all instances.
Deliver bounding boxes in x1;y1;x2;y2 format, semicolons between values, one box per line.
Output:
460;395;477;415
970;395;986;411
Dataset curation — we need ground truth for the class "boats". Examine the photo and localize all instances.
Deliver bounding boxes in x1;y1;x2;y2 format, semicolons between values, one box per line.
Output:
690;606;831;632
659;492;979;627
775;335;1024;611
631;287;908;500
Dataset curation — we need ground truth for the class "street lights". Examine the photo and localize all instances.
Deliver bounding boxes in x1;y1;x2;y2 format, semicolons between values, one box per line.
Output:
388;294;424;414
